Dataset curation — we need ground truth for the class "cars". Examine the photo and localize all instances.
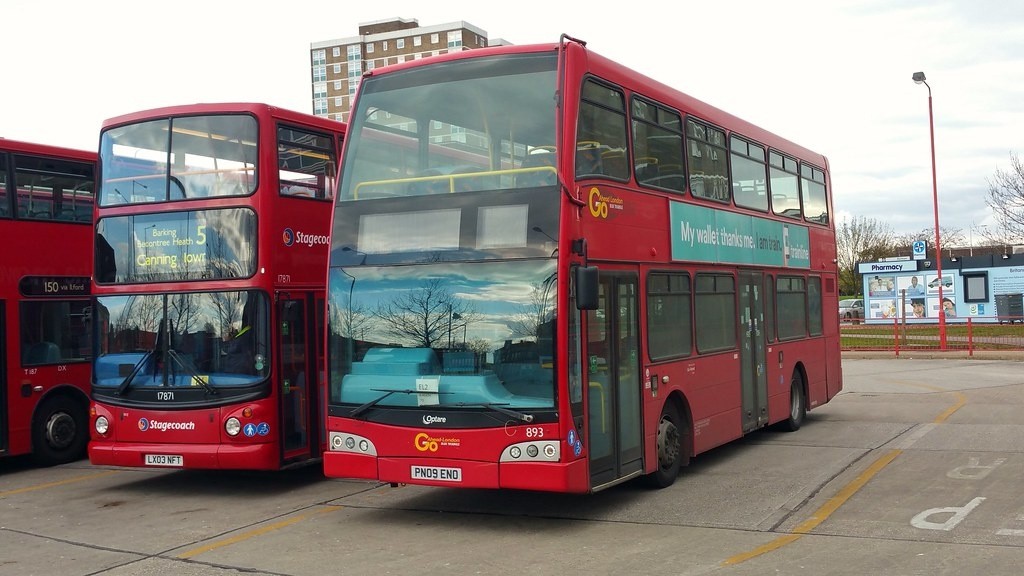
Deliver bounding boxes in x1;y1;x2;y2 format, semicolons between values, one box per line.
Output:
837;297;866;323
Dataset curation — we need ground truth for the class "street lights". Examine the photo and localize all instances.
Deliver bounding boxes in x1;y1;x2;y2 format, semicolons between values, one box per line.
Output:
911;71;949;352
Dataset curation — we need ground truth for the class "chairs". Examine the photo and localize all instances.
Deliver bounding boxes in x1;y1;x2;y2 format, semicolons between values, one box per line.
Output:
408;141;823;231
15;205;92;221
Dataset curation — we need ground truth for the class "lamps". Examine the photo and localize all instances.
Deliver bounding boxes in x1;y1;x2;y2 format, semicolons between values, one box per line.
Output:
1001;254;1010;260
950;256;959;262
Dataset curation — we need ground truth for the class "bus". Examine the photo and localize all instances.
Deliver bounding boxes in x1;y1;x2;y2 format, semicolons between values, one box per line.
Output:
1;137;317;465
323;33;843;495
87;102;521;469
